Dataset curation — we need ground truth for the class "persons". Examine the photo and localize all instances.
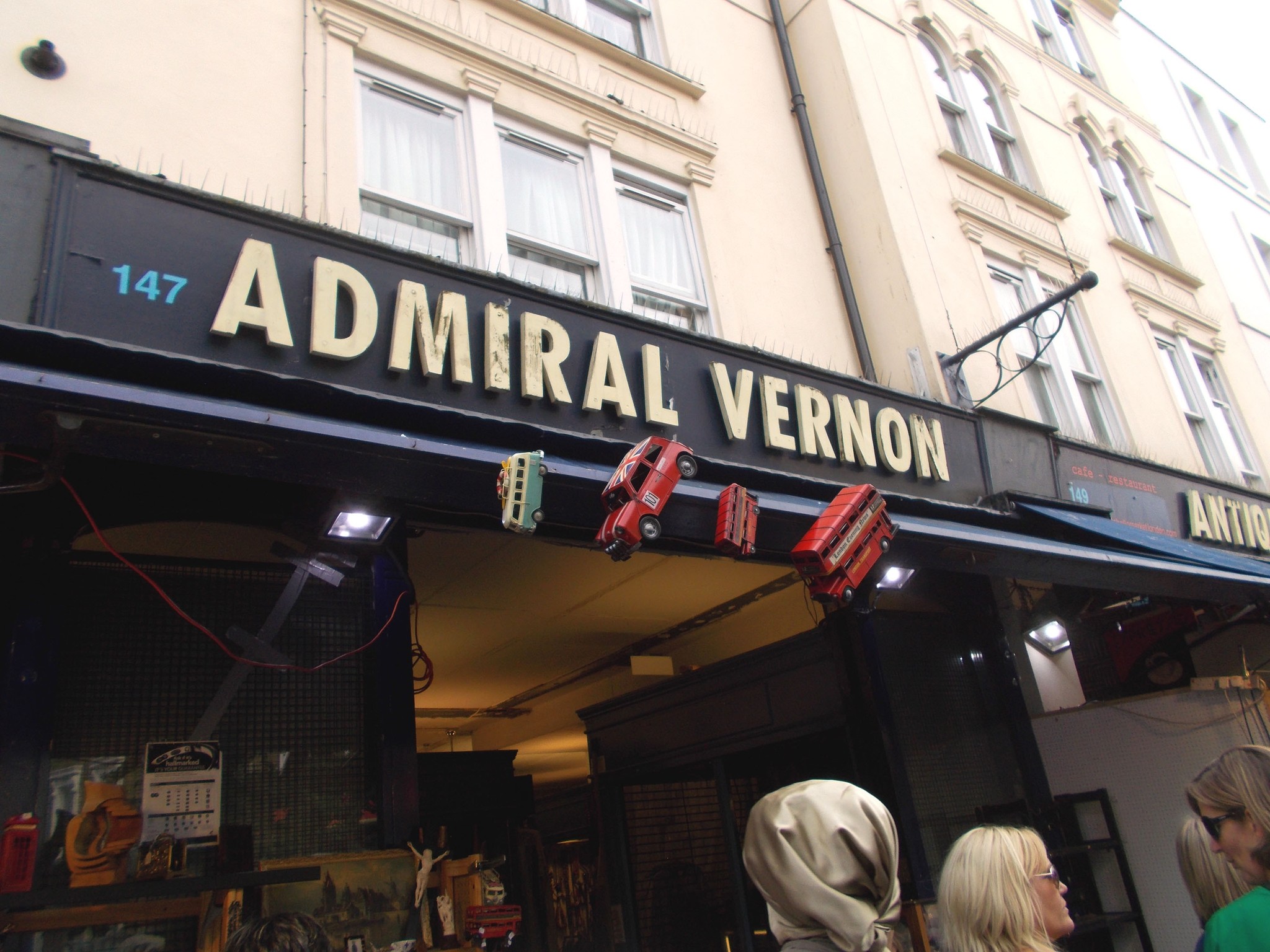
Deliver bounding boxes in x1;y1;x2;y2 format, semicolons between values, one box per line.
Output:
352;940;357;952
1176;745;1270;952
223;911;336;952
742;779;905;952
407;841;450;908
938;826;1075;952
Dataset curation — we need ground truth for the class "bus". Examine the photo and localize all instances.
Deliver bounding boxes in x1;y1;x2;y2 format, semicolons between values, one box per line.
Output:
716;482;760;558
464;906;524;941
494;452;548;539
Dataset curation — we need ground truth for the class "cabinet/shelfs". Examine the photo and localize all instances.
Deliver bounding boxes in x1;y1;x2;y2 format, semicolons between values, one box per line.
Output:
977;787;1153;952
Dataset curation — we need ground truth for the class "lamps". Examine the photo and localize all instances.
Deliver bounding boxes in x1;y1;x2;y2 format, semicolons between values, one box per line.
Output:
1022;597;1070;657
314;499;398;549
872;555;915;591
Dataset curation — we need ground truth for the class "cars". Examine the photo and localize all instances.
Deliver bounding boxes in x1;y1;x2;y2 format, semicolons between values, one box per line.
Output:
595;435;699;561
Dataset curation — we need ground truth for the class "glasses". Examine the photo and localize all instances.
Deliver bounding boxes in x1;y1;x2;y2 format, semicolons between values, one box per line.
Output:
1200;811;1234;841
1033;864;1059;883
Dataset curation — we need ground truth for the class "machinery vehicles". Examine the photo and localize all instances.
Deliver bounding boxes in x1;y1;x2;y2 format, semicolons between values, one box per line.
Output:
790;482;900;615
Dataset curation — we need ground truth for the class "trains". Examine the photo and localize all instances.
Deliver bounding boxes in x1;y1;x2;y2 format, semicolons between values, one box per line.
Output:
482;867;504;906
